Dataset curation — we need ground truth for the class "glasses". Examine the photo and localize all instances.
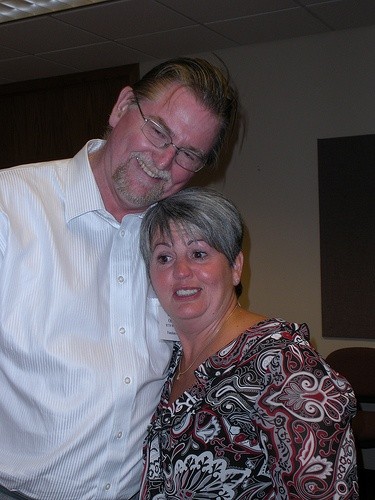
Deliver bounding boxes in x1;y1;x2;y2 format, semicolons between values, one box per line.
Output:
132;88;210;174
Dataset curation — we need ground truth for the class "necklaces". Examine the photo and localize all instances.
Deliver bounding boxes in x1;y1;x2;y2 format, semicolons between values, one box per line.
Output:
176;304;239;380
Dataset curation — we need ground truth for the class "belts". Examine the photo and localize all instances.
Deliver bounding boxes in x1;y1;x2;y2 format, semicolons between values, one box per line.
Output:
0;484;35;500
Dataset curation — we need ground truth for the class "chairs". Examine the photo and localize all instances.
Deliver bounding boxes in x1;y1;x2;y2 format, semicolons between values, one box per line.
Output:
325;346;375;480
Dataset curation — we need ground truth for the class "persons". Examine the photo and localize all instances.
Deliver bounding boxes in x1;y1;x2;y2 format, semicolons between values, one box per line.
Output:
0;52;238;500
138;187;359;500
